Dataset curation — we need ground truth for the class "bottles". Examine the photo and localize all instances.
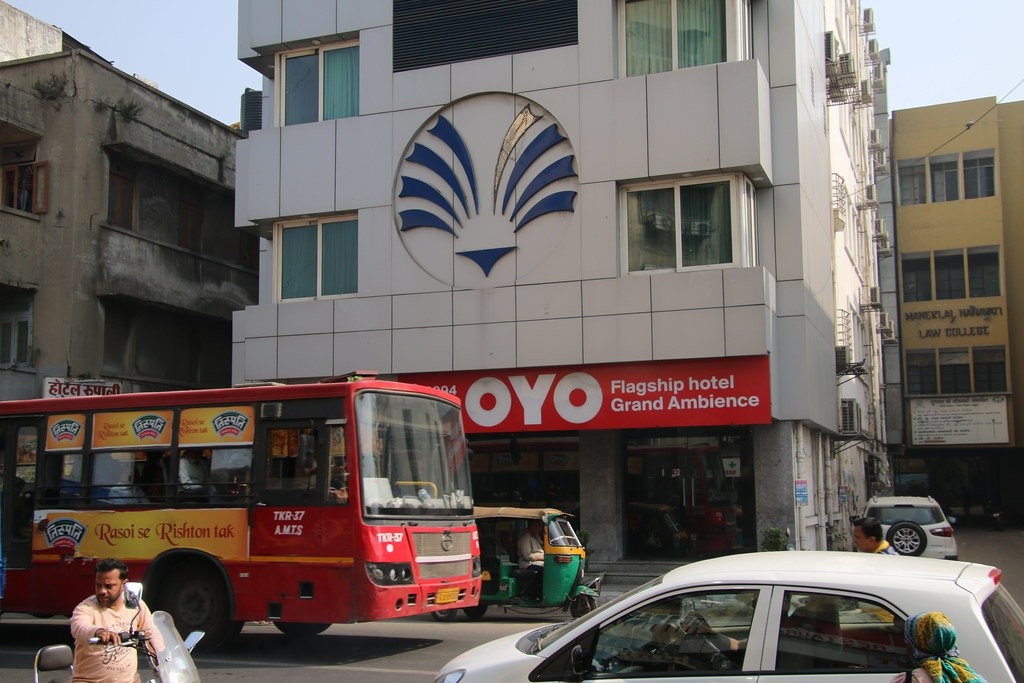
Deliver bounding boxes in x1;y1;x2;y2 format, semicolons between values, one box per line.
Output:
418;489;435;508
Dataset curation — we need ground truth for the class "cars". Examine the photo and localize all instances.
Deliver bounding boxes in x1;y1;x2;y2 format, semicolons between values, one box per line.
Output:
432;550;1023;683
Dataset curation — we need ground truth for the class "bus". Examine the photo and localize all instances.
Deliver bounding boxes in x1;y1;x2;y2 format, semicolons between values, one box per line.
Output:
1;371;483;656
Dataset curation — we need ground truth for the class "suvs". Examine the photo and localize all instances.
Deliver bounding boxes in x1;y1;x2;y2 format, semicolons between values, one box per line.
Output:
849;495;958;561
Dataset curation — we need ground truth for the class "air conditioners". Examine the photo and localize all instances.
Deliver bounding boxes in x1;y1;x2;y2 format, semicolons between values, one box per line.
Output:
866;183;877;206
876;218;890;256
869;38;878;60
880;312;895;343
861;80;872;103
870;286;882;310
649;211;672;233
871;128;888;175
836;345;852;370
841;398;863;434
690;222;710;236
864;8;873;32
824;31;857;102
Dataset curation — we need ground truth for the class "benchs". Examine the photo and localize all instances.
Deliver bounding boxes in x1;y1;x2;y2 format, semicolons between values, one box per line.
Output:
477;557;501;594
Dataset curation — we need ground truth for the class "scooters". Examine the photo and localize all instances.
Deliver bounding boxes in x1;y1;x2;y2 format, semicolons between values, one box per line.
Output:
33;586;205;682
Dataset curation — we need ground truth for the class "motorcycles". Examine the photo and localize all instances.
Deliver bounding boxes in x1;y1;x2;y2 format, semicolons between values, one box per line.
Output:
431;508;602;619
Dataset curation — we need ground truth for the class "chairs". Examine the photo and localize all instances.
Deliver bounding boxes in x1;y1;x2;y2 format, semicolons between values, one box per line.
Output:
778;598;844;668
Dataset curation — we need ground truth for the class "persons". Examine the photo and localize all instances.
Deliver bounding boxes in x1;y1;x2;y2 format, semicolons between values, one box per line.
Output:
14;449;250;504
853;517;901;556
889;612;990;683
681;591;792;670
70;559;166;683
517;518;545;601
293;429;337;492
